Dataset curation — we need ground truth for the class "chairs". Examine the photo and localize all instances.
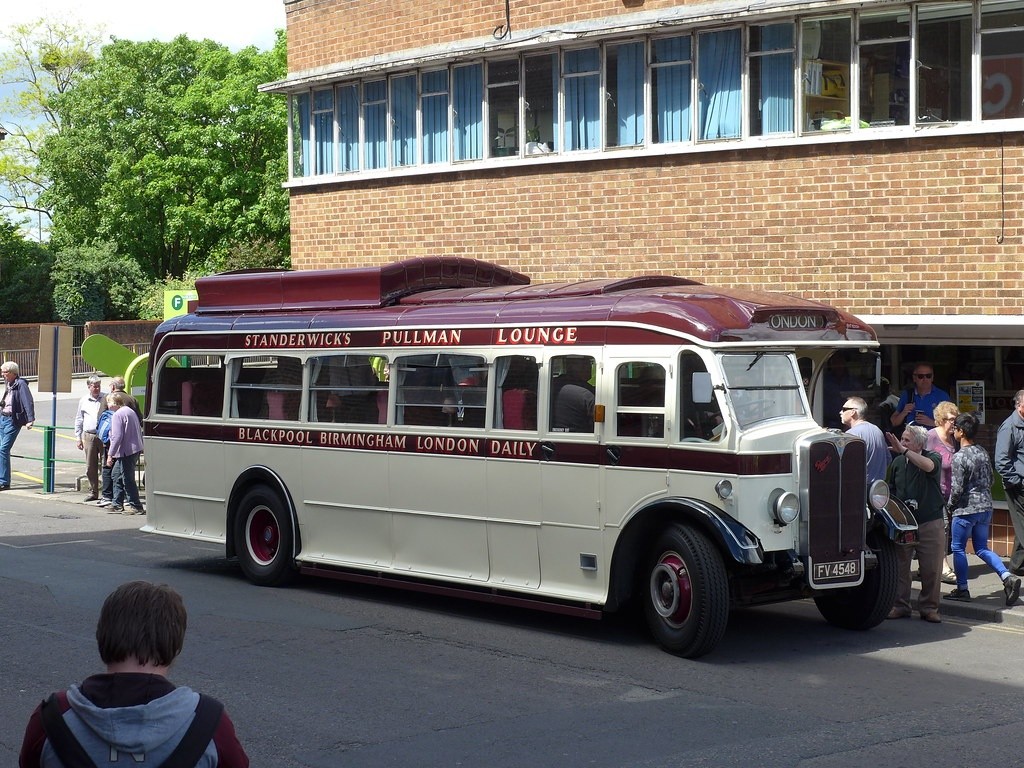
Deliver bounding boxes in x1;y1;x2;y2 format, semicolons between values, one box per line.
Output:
180;374;537;431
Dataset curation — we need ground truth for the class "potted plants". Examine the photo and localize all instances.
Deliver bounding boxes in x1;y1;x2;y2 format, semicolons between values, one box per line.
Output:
494;125;518;157
526;126;544;153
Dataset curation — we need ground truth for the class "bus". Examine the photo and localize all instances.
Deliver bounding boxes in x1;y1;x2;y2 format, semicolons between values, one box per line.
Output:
139;254;919;657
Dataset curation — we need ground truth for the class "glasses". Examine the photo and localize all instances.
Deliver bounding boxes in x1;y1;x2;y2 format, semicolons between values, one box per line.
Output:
843;406;859;412
914;374;933;379
943;419;954;424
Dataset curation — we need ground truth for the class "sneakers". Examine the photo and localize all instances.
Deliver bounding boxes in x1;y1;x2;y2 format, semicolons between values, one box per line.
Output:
1003;574;1021;605
943;589;970;602
123;505;144;515
106;504;125;514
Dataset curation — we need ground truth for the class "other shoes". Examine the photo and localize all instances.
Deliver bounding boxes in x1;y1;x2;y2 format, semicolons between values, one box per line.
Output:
95;497;113;507
84;495;97;502
0;483;10;491
887;610;911;618
921;610;941;623
941;569;957;583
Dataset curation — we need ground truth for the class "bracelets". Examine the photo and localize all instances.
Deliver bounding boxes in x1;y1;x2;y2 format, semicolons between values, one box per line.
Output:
903;449;909;456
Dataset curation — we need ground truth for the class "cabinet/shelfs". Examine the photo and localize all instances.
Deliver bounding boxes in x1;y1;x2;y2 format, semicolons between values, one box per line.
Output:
802;34;954;132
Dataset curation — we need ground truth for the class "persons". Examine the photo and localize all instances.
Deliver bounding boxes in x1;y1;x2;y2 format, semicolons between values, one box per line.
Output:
105;394;145;515
839;362;1024;623
867;377;907;460
75;376;109;502
0;361;35;491
319;354;723;439
18;581;250;768
96;377;144;506
